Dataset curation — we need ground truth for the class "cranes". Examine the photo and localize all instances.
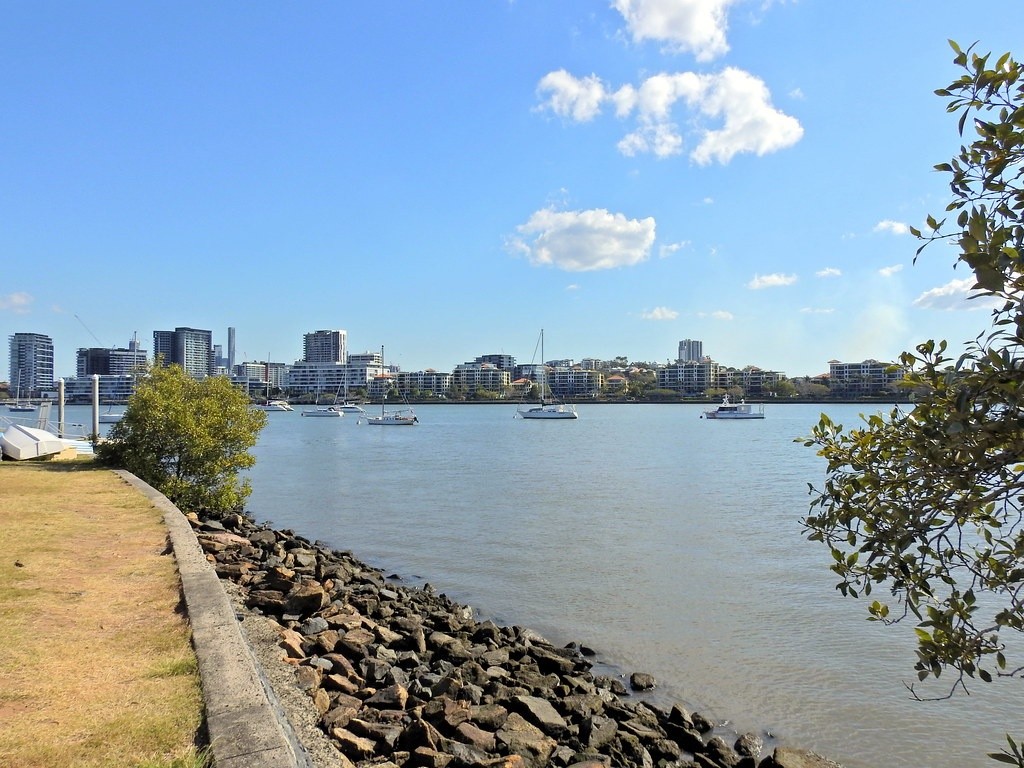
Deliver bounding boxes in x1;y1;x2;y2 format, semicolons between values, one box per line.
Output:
74;315;106;348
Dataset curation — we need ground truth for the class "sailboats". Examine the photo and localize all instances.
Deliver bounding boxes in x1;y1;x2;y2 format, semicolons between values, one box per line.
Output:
98;329;138;423
333;361;366;414
366;344;419;425
513;328;579;419
5;369;39;412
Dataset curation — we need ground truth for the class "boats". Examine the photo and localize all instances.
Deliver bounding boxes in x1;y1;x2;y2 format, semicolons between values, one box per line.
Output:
255;399;295;412
301;406;345;417
702;393;766;420
